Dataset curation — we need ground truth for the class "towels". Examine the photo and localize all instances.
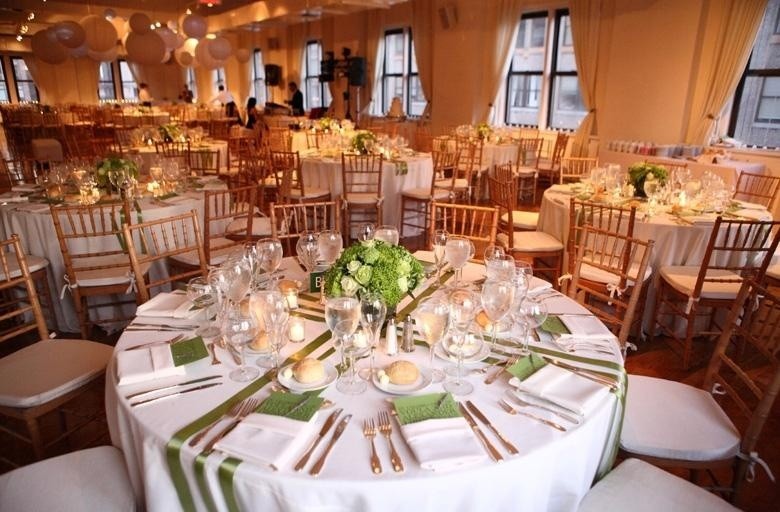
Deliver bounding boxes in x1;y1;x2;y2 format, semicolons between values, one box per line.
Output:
677;210;716;226
549;182;583;193
525;272;552;296
114;335;209;388
195;178;226;192
11;182;44;193
212;390;323;468
616;206;647;221
410;246;437;268
135;290;214;320
507;352;610;416
159;192;196;205
536;312;614;345
732;200;768;209
394;391;488;469
728;206;769;220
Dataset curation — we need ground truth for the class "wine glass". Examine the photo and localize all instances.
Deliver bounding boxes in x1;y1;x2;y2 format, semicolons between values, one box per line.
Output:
481;277;515;355
256;238;283;290
415;296;450;384
247;286;285;368
360;292;387;383
483;245;505;272
590;167;602;197
296;230;320;287
513;295;548;356
108;170;127;201
513;261;533;277
223;311;261;383
443;289;475;398
186;275;221;338
644;178;658;213
166;162;179;192
357;222;374;244
430;229;448;289
445;235;471;291
364;138;374;155
150;165;163;200
660;167;738;218
258;312;294;391
332;318;375;396
515;273;530;309
207;270;235;335
244;242;263;293
375;225;399;247
220;257;252;323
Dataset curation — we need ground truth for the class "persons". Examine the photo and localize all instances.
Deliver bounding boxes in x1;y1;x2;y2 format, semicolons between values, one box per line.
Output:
180;83;192;101
208;86;236;116
283;82;305;116
245;96;259;131
139;82;152;107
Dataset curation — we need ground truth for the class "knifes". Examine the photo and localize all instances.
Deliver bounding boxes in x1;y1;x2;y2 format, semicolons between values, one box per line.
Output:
542;354;622;384
125;328;177;331
131;382;223;406
310;414;353;475
131;323;200;329
543;312;600;318
466;400;518;454
125;375;222;399
457;401;503;462
293;254;308;274
294;407;343;472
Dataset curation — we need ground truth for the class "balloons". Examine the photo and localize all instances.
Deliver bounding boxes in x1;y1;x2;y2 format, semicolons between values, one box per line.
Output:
27;12;240;70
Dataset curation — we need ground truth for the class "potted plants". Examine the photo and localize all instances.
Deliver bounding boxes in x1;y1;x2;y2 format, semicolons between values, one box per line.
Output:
628;162;668;198
157;125;182;144
320;117;331;132
322;239;425;338
96;158;138;195
477;124;494;142
351;131;376;154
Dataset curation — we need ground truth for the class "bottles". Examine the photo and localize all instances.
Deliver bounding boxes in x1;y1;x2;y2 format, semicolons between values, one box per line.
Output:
400;314;416;353
385;318;398;357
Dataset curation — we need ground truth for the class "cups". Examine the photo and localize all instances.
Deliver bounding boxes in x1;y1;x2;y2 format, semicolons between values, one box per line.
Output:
469;243;476;259
324;292;362;376
486;255;514;287
317;230;343;263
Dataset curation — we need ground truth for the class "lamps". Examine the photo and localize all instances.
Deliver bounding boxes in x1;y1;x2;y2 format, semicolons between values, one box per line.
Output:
0;8;34;41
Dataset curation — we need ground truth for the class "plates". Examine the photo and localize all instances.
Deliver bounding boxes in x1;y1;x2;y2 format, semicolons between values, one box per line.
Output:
432;338;490;364
372;371;434;395
277;362;339;392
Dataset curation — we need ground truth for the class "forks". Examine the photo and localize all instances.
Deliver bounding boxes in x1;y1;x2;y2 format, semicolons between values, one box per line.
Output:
485;357;518;384
504;389;579;424
361;416;382;474
207;343;221;366
203;398;258;454
188;399;244;447
377;410;403;472
124;333;184;351
496;398;567;432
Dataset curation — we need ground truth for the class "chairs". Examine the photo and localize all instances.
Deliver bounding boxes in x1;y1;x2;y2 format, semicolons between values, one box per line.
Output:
400;137;485;249
49;200;151;340
578;457;744;511
531;132;570;202
499;138;543;210
488;170;564;290
121;209;207;304
0;252;62;337
269;199;341;243
452;161;518;250
225;168;292;244
428;200;499;262
269;150;331;238
339;152;382;248
650;217;780;371
567;199;655;360
0;446;136;512
520;129;539;152
0;234;115;475
167;186;257;291
732;171;780;211
620;275;780;507
560;157;598;184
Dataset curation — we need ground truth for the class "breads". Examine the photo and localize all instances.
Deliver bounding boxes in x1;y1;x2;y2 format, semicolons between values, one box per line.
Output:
248;329;269;351
284;358;326;383
378;360;420;386
48;185;59;199
239;298;249;317
279;279;301;294
476;309;499;329
628;199;639;209
63;193;76;203
595;192;603;202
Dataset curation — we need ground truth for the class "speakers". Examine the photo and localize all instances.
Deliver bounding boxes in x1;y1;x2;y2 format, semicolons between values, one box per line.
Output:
264;65;280;85
319;61;335;82
348;57;367;85
